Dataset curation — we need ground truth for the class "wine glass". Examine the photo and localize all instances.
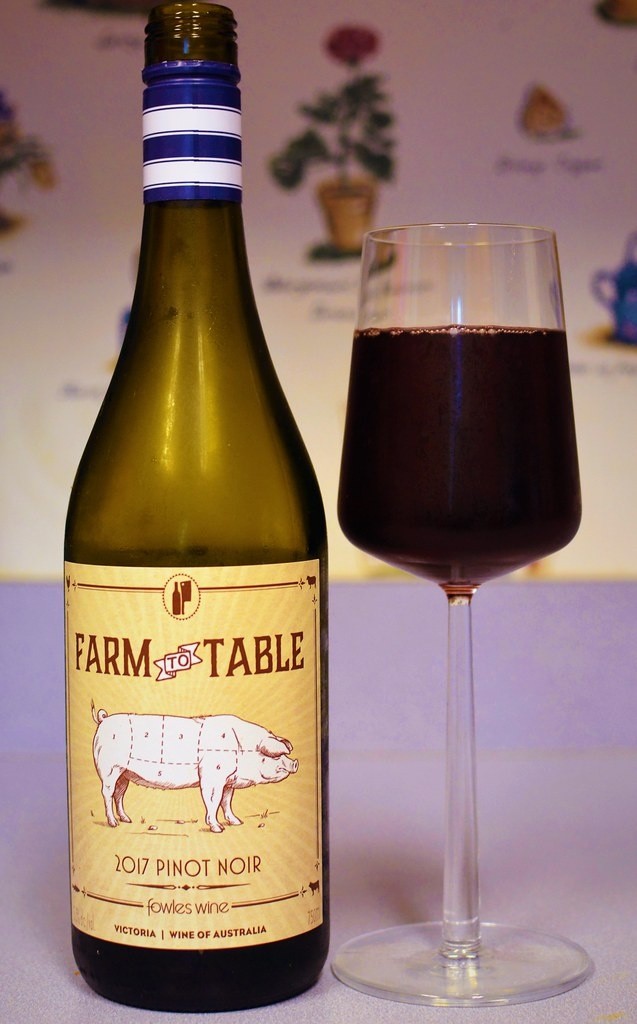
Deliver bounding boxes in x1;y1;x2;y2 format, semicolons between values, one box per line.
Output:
331;222;593;1007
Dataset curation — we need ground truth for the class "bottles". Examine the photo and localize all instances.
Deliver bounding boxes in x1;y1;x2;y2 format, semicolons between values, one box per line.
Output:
61;3;331;1014
